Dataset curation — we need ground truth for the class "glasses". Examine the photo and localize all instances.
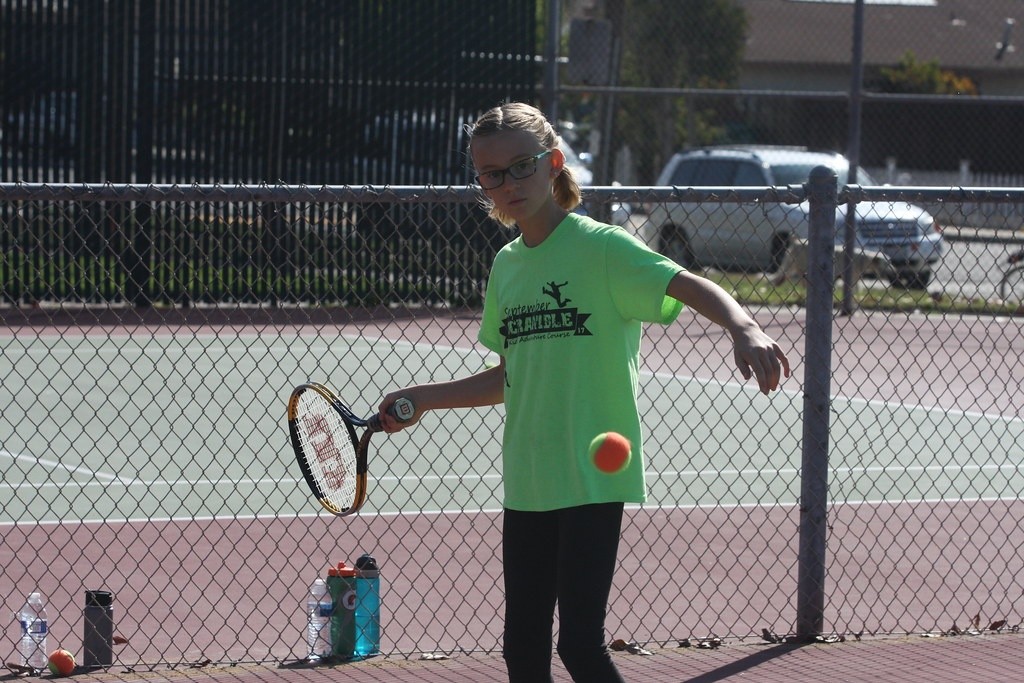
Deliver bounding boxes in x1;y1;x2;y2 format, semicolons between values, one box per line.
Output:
473;149;553;190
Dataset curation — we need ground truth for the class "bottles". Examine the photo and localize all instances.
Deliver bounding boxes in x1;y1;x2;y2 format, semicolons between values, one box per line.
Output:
21;592;47;668
306;578;332;660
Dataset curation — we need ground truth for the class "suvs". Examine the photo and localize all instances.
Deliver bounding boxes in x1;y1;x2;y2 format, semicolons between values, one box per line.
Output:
646;143;944;292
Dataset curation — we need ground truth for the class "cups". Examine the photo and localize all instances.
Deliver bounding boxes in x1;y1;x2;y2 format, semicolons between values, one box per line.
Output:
83;590;113;668
327;553;381;660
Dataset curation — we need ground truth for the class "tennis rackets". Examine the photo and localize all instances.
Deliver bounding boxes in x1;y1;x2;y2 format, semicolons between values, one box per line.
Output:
287;381;416;517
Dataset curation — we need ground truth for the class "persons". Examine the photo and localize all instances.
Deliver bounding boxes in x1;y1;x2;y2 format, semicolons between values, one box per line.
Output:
376;103;791;683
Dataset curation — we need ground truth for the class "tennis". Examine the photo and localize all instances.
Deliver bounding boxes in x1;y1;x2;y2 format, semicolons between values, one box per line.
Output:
589;431;632;476
48;649;75;676
